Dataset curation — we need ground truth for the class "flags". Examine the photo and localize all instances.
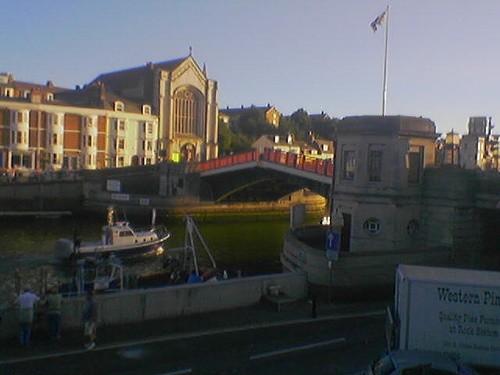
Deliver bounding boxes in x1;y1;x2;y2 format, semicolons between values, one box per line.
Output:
369;11;388;32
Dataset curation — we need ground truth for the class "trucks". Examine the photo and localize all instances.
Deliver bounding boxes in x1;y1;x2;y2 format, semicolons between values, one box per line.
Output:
384;265;499;368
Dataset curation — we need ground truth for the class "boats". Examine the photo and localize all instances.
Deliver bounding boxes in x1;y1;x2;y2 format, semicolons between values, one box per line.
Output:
56;206;171;266
74;214;228;294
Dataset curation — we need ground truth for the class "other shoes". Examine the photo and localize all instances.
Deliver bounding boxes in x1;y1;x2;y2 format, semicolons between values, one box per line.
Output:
84;341;93;347
88;342;96;349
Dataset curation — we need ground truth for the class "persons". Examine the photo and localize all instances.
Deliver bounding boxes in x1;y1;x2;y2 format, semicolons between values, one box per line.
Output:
77;282;99;352
13;287;41;351
40;283;64;346
183;261;229;283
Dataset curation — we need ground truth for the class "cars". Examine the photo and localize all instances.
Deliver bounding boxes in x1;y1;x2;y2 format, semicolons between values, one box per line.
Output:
354;348;475;374
